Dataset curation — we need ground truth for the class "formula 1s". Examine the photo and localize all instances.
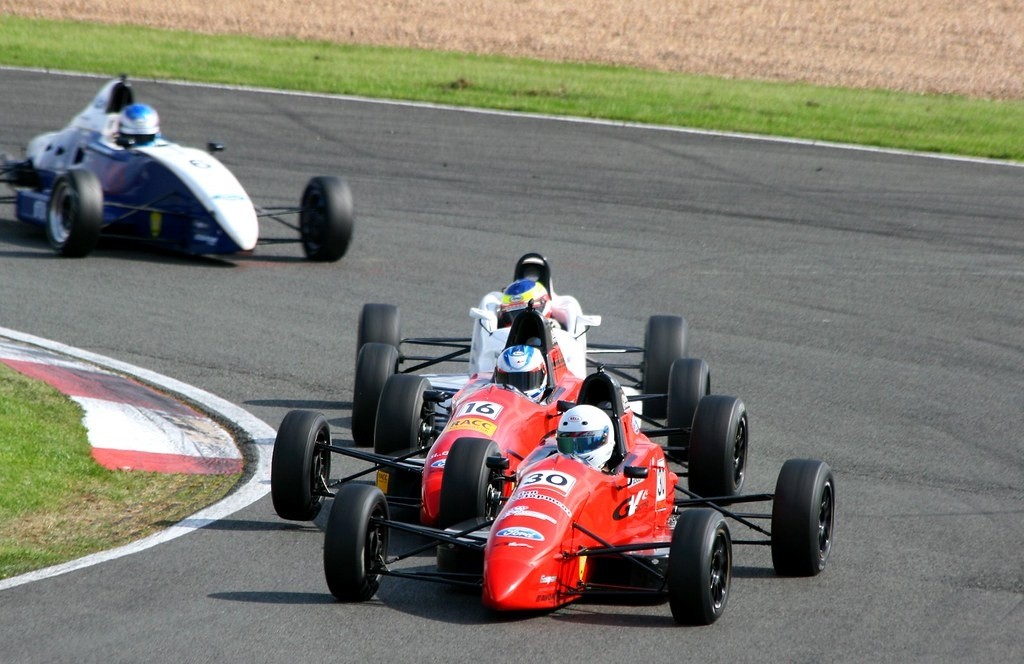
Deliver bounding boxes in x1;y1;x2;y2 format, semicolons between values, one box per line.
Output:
1;71;356;265
269;250;836;628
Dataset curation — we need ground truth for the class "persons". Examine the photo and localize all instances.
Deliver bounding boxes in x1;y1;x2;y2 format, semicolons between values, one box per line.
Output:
556;404;616;475
497;279;569;331
494;344;548;406
115;102;162;150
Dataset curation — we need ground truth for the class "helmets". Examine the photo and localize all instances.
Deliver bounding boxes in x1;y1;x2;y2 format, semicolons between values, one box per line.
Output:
500;281;550;327
555;404;615;469
117;103;161;149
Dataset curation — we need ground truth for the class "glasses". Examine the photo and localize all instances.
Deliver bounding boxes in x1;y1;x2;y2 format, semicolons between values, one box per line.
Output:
556;435;603;456
494;345;547;402
